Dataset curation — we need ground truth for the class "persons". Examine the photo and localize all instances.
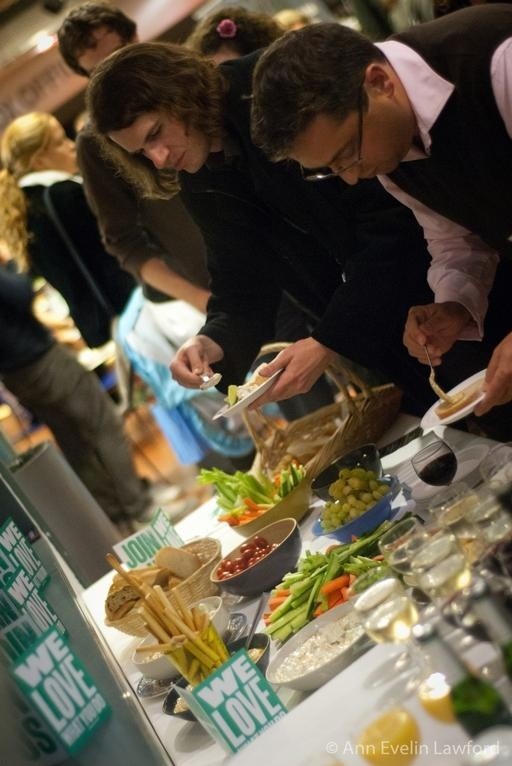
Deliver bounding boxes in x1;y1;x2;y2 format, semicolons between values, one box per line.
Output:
249;0;512;415
0;237;187;522
0;108;136;351
184;3;290;67
85;41;472;412
55;0;215;313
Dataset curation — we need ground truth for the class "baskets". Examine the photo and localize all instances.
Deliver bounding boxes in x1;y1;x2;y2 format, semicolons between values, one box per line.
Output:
237;340;404;479
101;533;225;641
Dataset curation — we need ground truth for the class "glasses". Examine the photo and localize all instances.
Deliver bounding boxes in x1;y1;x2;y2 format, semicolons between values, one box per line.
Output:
297;99;378;186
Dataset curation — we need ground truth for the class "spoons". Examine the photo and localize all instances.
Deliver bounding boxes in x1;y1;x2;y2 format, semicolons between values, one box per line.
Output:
196;367;222;389
419;334;456;402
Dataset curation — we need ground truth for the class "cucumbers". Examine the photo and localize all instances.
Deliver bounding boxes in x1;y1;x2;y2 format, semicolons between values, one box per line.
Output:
263;510;425;645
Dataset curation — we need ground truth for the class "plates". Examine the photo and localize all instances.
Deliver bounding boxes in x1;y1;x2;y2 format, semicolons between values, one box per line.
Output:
136;609;247;698
420;366;489;432
208;366;284;424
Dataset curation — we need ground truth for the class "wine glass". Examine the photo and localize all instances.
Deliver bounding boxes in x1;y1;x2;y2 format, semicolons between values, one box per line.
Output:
349;437;512;766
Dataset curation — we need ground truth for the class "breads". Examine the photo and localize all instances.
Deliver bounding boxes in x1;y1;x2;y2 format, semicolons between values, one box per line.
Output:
105;545;201;621
434;377;485;420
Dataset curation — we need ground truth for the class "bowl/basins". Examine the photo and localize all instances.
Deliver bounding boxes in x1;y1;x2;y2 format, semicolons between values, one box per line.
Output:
210;515;302;600
314;473;403;543
162;632;270;721
130;595;229;679
265;582;386;694
313;440;386;507
225;463;315;543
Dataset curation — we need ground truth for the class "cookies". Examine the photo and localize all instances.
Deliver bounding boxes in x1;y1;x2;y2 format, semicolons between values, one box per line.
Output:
106;552;230;687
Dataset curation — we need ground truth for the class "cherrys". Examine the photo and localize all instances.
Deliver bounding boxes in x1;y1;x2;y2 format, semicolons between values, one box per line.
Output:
217;535;274;580
